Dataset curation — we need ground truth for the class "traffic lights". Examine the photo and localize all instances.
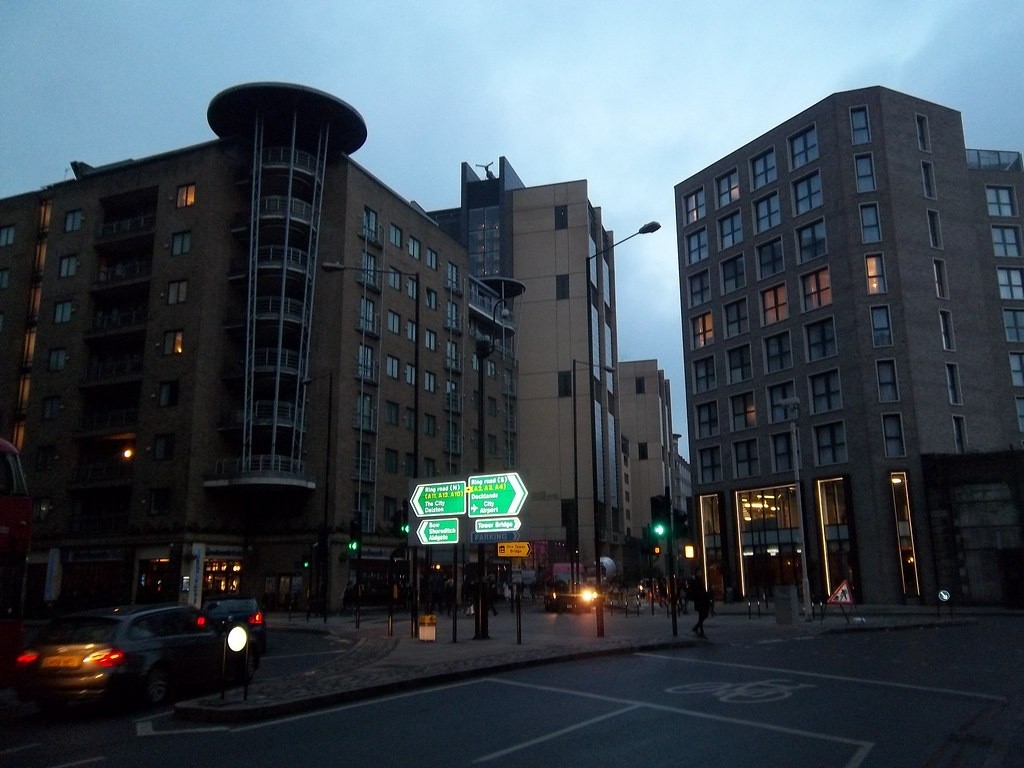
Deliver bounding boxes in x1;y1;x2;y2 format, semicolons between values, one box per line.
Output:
348;512;363;553
301;552;309;569
389;499;409;539
649;495;669;540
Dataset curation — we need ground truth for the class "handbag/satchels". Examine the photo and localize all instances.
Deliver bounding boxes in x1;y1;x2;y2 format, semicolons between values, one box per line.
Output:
685;586;694;601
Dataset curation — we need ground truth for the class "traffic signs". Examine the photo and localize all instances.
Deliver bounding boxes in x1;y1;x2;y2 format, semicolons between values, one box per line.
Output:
416;517;459;544
475;517;522;532
409;481;466;518
466;473;527;519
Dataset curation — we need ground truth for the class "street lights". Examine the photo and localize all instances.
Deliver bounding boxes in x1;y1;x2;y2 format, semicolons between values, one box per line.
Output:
585;220;661;638
321;260;420;638
572;357;615;613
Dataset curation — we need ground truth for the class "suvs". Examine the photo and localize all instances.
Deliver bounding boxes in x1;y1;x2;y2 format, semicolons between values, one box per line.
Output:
16;603;254;716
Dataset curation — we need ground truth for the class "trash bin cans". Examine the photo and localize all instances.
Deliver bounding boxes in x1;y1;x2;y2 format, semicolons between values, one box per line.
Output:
419;615;437;643
774;586;799;625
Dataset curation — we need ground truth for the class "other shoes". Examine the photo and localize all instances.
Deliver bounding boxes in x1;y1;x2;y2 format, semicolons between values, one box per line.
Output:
692;627;699;635
699;632;708;639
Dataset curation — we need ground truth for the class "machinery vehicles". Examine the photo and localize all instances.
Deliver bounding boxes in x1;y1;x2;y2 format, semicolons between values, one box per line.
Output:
548;556;617;612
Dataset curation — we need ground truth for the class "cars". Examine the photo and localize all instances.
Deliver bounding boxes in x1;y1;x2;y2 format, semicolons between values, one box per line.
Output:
202;595;267;655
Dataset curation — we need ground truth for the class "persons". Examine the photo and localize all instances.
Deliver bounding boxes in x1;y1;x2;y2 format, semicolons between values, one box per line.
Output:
442;576;455;615
709;584;715;618
487;573;499;616
690;568;711;639
460;574;478;616
554;575;567;591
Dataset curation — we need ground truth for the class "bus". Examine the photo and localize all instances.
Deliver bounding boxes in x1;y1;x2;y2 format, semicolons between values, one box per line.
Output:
0;440;35;692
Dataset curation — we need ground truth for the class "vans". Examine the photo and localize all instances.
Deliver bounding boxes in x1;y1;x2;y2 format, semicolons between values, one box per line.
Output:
638;578;660;599
